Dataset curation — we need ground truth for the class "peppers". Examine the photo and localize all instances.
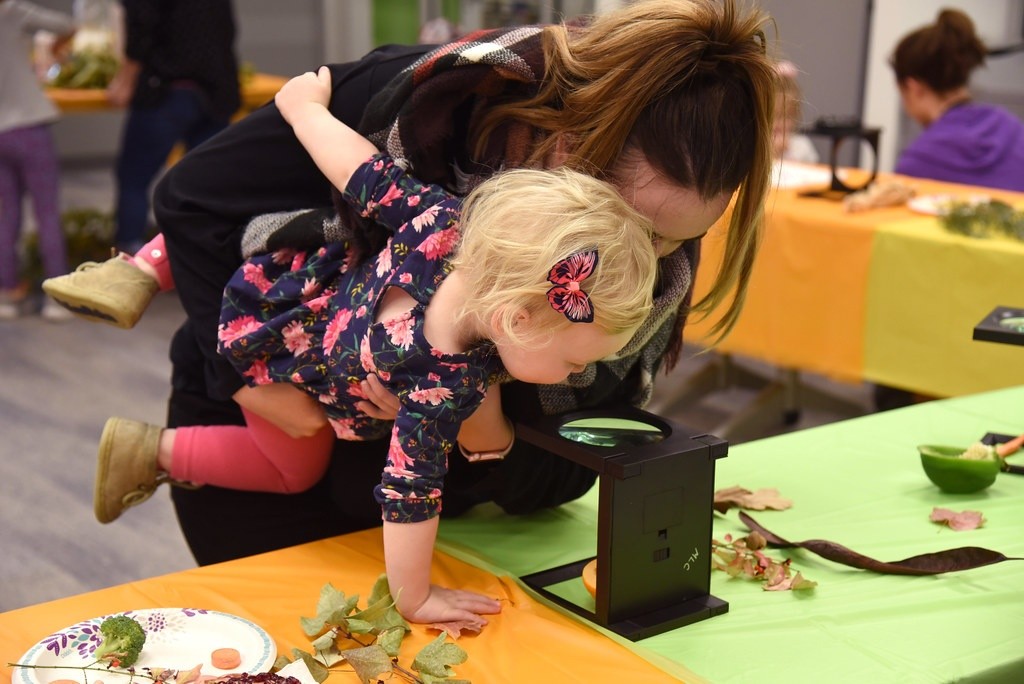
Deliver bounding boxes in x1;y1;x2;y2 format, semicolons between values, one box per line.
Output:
917;442;1000;493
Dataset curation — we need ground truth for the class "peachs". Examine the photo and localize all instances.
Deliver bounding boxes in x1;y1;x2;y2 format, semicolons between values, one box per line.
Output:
582;557;596;597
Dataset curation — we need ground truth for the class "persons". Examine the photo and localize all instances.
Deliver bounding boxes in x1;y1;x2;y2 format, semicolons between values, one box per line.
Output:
0;0;839;628
886;7;1024;197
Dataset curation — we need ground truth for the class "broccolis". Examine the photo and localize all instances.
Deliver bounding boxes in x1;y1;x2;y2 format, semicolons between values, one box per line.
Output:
97;615;145;668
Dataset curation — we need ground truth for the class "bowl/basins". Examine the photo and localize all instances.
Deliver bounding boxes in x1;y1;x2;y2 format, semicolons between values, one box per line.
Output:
918;445;1003;494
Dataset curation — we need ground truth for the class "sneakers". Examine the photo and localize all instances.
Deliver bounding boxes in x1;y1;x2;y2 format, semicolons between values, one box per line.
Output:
94;417;197;524
42;247;160;330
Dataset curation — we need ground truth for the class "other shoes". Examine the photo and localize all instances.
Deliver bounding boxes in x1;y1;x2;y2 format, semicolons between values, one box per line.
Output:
0;290;43;318
43;302;75;321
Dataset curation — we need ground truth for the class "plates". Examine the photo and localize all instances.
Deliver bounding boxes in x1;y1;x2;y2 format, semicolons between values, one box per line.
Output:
12;608;277;684
908;194;990;215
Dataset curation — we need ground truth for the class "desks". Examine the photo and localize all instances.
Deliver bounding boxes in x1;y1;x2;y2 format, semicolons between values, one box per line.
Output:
685;163;1024;423
1;375;1024;684
37;67;293;165
